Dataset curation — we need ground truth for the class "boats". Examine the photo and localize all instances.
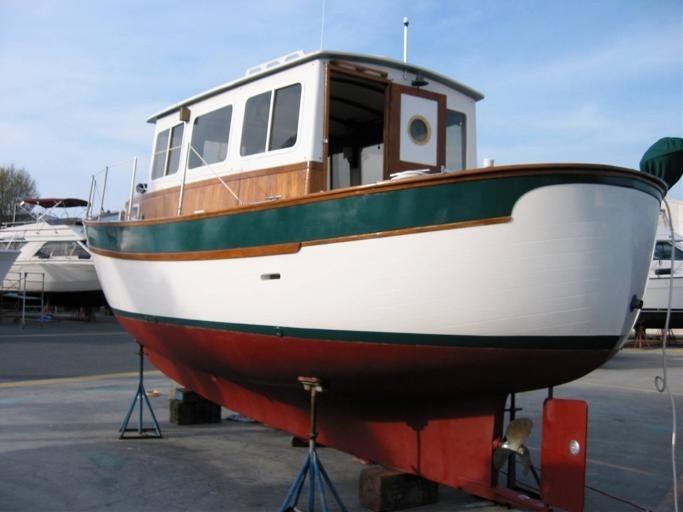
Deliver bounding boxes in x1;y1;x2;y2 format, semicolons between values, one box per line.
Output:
0;195;103;306
631;207;683;329
80;16;683;510
